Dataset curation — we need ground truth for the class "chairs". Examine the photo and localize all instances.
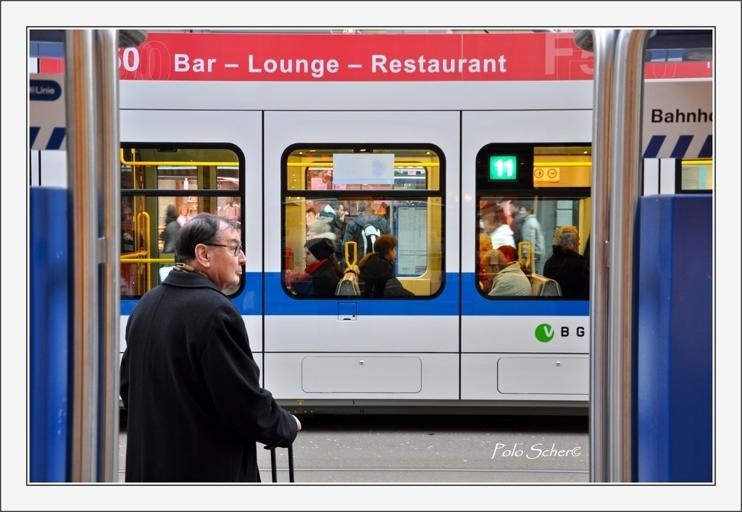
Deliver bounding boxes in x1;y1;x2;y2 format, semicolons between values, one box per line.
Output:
536;273;563;297
335;263;362;298
529;276;548;297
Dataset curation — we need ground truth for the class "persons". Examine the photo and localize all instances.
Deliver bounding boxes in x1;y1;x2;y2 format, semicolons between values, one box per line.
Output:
121;188;589;299
120;212;301;482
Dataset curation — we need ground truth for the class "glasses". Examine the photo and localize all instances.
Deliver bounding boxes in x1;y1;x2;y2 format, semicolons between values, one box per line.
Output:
205;242;243;257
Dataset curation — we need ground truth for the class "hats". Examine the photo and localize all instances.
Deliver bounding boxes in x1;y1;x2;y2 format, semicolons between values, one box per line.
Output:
305;237;335;262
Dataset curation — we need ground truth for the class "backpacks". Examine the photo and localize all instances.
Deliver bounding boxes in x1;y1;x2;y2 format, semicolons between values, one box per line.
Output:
356;223;384;258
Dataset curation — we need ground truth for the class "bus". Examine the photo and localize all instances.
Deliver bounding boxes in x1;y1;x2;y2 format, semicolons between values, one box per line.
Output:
29;31;713;425
